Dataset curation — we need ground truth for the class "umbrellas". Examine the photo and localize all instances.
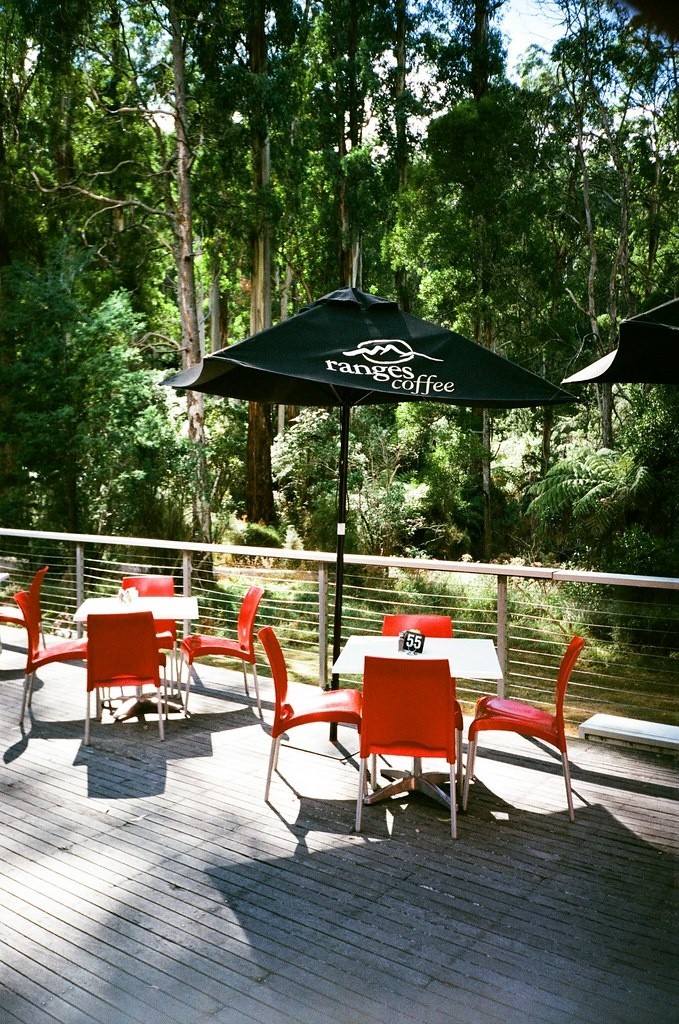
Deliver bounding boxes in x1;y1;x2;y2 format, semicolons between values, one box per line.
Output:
559;294;678;392
157;287;583;745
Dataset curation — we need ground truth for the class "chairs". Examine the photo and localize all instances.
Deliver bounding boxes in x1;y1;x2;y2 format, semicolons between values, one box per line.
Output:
456;633;589;823
380;613;456;640
0;564;53;665
252;628;378;810
120;572;178;598
354;657;467;837
174;584;267;716
83;613;170;744
14;591;93;727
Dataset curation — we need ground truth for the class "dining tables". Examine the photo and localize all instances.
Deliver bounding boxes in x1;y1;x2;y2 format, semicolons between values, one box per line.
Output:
74;595;201;719
331;636;498;813
